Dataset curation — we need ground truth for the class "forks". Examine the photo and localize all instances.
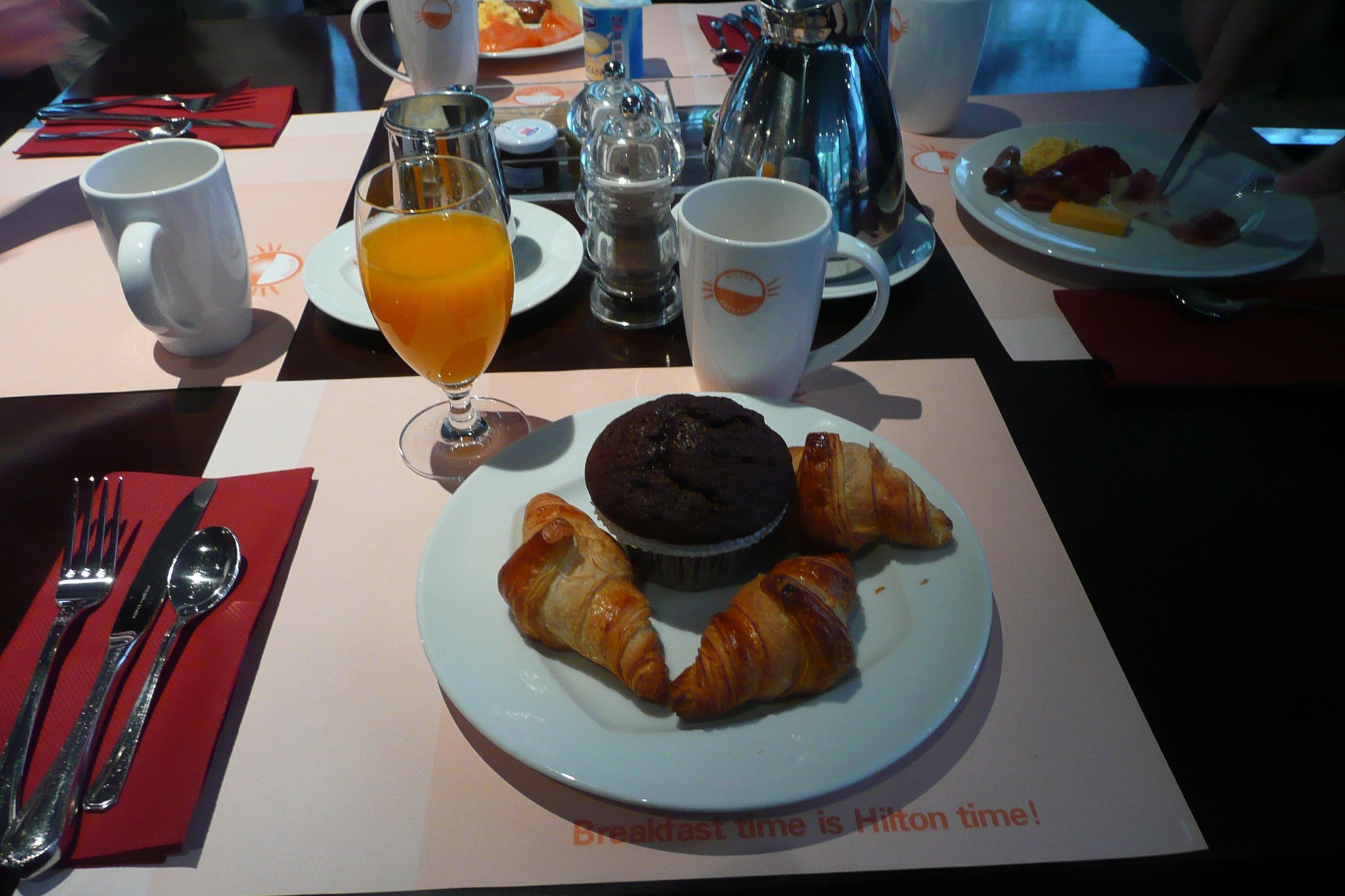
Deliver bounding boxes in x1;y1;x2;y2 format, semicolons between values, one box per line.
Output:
1;477;123;896
49;75;251;115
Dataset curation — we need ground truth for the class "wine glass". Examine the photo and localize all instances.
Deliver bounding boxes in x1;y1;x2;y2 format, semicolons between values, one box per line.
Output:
352;154;534;482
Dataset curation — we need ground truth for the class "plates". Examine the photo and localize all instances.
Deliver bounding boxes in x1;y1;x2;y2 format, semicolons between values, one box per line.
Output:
669;195;935;300
949;122;1316;280
301;197;584;331
477;1;585;60
415;388;996;816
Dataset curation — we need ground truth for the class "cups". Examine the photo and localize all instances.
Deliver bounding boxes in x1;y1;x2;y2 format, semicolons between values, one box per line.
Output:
574;0;653;81
888;1;994;136
383;82;518;246
676;177;889;405
78;137;252;358
350;0;478;96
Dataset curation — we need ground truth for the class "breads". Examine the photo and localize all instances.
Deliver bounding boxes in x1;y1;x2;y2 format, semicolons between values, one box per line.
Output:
467;383;962;732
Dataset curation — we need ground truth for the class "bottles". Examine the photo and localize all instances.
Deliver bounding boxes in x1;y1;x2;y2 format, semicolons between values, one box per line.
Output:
563;60;667;222
494;115;560;194
576;95;684;328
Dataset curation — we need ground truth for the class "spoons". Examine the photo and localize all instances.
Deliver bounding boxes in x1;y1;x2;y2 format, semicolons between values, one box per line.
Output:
35;118;194;142
81;525;241;812
709;19;741;59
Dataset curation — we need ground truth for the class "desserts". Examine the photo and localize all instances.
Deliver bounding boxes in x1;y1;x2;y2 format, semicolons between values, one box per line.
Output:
976;118;1236;260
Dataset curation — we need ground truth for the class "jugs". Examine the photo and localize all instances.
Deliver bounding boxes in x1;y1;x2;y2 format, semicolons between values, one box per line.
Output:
708;0;906;254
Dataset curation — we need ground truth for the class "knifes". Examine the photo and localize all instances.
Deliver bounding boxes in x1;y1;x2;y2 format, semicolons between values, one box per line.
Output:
1;479;220;867
1159;72;1236;194
33;109;276;129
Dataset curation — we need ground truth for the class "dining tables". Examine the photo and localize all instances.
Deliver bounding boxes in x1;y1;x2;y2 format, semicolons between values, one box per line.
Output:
2;0;1345;894
